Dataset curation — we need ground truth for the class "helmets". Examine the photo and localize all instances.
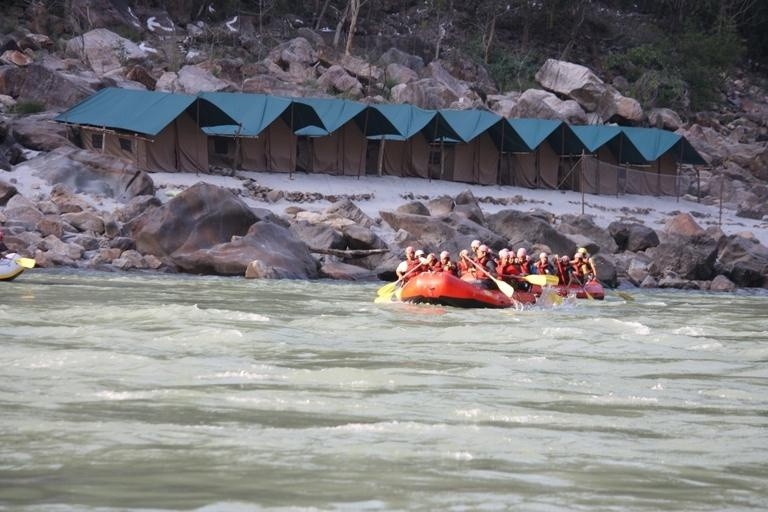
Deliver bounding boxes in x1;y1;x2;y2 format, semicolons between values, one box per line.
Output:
405;239;588;263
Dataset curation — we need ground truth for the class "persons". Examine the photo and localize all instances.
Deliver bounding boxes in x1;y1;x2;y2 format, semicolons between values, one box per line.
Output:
389;239;597;291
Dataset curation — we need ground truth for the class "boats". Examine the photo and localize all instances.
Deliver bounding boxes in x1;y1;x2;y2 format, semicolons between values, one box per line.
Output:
374;272;604;309
0;253;24;280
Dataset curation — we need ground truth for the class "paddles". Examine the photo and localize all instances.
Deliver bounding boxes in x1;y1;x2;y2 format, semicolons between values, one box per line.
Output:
594;278;634;301
571;269;595;301
373;281;407;304
461;253;514;298
0;256;36;269
490;271;547;286
541;285;562;306
376;260;424;298
518;273;560;286
